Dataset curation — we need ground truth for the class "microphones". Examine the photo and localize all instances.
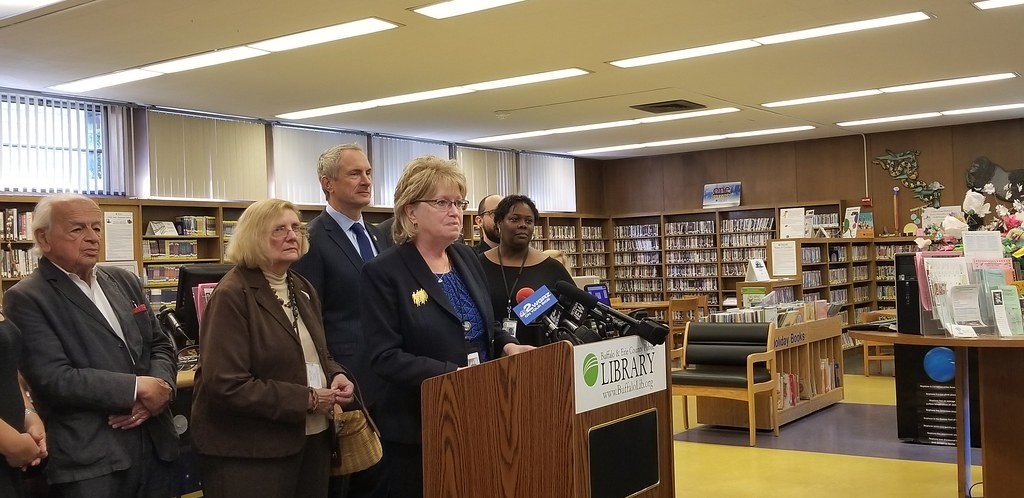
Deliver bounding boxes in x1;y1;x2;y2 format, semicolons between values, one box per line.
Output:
512;280;670;347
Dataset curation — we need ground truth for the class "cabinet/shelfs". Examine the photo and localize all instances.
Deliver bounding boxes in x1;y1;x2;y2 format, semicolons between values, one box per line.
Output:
766;236;937;352
1;193;847;316
696;320;844;432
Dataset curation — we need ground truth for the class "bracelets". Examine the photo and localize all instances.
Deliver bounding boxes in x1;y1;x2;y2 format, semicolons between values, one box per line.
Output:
25;408;39;416
307;387;320;415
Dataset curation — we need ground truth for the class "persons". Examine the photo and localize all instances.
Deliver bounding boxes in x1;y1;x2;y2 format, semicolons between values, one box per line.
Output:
2;192;182;498
376;217;465;249
478;195;577;347
0;310;48;498
357;155;540;498
190;198;355;498
473;193;504;255
290;143;395;498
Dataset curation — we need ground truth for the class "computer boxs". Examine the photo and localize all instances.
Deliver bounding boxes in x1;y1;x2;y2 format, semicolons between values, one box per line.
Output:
893;253;944;337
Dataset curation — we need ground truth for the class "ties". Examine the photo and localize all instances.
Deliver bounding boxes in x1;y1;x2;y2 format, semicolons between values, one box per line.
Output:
350;223;375;262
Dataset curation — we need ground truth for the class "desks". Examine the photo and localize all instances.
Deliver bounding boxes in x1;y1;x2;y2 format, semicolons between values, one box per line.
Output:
613;300;669;321
847;329;1024;498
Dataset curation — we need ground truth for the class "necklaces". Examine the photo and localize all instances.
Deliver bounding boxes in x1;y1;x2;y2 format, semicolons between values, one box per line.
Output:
267;276;299;337
497;246;530;322
416;246;447;283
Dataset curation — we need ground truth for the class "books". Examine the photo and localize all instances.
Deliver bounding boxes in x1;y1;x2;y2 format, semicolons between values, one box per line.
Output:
0;209;941;410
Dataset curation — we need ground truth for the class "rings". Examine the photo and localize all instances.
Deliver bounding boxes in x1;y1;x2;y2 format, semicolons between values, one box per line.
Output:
132;416;136;421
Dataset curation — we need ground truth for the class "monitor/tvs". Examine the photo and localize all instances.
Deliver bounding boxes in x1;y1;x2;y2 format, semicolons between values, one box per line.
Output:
173;263;237;352
584;284;611;308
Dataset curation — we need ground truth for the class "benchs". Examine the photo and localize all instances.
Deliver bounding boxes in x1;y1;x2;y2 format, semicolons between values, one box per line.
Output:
671;321;779;446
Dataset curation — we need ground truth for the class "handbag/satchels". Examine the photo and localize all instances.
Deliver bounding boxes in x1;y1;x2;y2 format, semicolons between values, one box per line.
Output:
331;361;383;476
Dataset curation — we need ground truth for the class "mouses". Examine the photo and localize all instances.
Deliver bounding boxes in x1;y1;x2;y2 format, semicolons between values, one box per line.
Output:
878;323;896;332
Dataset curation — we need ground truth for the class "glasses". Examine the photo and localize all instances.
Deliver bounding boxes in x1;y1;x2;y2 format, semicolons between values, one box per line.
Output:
273;225;306;237
414;199;470;210
481;210;495;219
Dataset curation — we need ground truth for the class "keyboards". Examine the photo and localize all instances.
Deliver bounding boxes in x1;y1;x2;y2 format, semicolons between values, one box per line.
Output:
850;318;896;330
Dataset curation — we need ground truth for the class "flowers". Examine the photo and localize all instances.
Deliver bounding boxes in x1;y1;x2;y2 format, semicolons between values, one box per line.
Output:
914;183;1024;258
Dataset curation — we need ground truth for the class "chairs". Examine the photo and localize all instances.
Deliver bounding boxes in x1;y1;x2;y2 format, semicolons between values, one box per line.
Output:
668;294;709;350
862;311;896;376
